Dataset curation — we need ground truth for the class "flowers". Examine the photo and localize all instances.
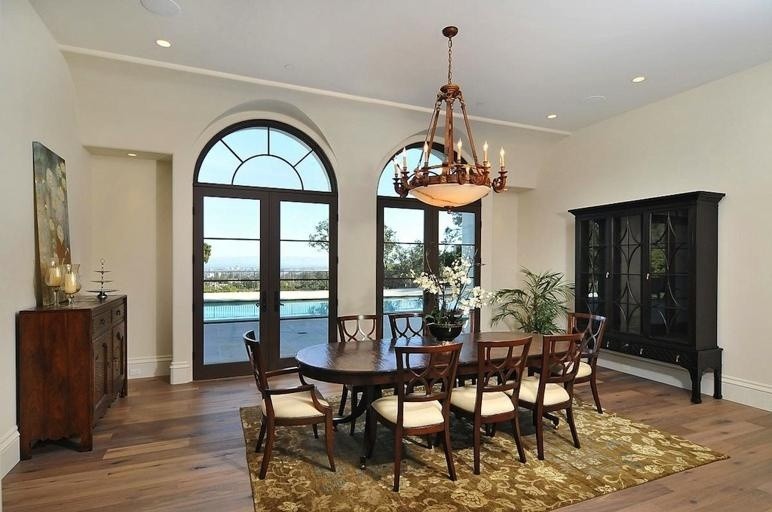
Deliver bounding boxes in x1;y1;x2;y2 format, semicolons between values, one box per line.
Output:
404;243;503;325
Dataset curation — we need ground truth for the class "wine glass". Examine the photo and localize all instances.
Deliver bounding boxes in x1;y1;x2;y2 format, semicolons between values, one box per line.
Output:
47;257;66;310
63;263;83;309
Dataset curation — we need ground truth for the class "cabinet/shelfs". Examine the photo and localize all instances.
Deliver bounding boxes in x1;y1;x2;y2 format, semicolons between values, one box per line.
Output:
17;295;132;461
566;189;726;404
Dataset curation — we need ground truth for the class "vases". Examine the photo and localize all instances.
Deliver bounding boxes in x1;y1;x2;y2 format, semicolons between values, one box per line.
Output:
426;323;465;342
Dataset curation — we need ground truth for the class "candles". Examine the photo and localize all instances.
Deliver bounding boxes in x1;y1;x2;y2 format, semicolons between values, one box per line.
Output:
47;266;61;287
63;273;77;294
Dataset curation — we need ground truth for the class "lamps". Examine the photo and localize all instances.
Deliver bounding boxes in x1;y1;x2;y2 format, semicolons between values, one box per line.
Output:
391;26;510;214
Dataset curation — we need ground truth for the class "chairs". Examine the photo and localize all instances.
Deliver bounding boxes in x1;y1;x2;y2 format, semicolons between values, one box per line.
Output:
242;329;337;479
388;313;446;395
436;336;533;475
491;333;585;460
366;341;464;492
334;314;399;438
546;312;609;415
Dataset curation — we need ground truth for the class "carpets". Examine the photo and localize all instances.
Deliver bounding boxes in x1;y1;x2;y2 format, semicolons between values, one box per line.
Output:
237;377;731;512
